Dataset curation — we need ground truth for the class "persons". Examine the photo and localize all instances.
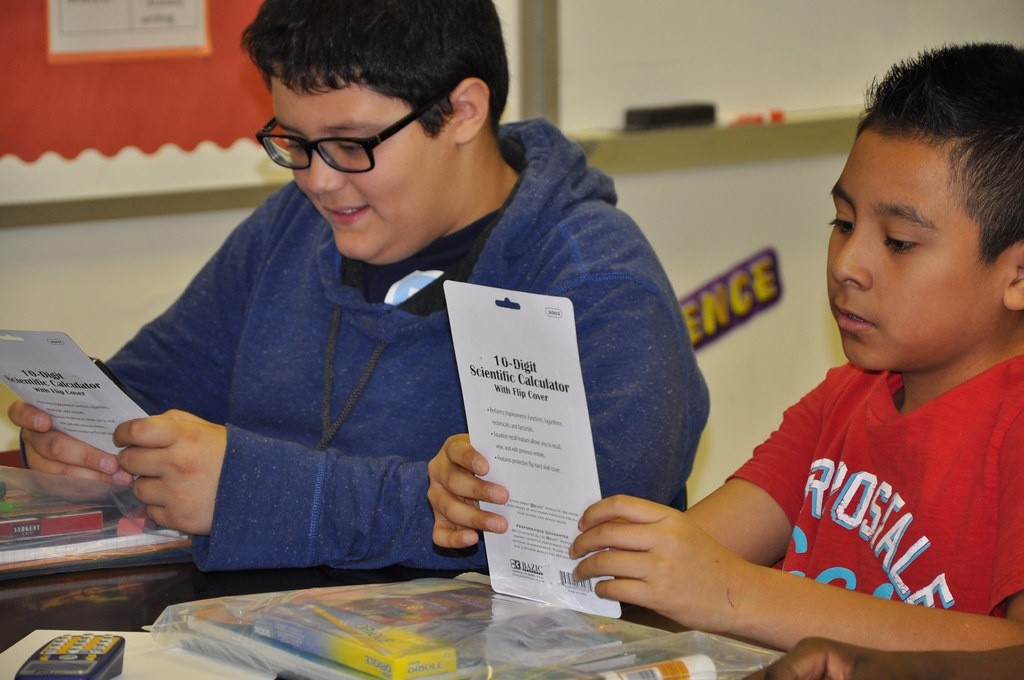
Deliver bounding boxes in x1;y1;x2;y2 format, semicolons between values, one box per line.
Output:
566;40;1024;649
6;0;710;632
740;637;1024;680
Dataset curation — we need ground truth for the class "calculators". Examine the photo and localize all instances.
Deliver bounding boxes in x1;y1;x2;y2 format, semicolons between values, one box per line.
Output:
13;634;126;679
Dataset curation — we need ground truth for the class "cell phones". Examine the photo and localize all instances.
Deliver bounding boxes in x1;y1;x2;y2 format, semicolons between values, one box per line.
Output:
16;633;125;680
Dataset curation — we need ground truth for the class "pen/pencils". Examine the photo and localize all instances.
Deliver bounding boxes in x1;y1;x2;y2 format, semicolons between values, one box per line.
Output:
0;501;70;512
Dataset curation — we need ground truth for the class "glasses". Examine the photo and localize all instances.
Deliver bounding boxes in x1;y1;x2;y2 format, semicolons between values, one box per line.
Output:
256;87;452;174
